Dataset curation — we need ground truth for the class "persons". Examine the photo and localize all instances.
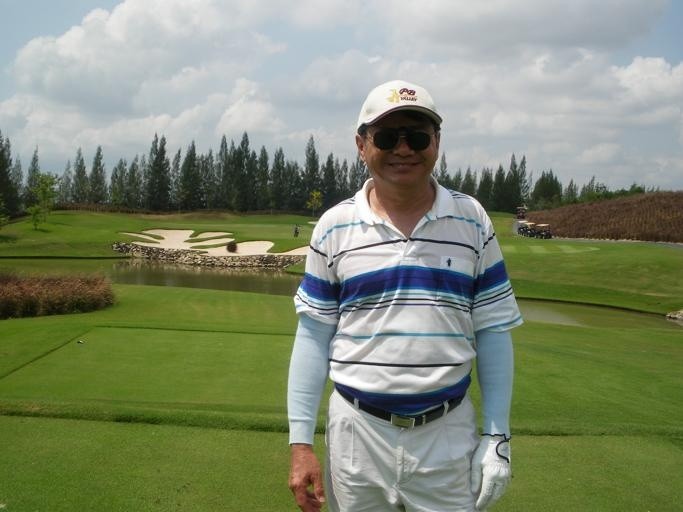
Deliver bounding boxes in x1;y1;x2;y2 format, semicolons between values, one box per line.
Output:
286;79;525;512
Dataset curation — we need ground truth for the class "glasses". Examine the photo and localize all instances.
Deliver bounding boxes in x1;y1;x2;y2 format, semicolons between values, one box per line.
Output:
371;129;430;150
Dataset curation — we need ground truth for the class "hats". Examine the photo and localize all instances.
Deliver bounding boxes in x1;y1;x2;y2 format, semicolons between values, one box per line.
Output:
354;80;442;134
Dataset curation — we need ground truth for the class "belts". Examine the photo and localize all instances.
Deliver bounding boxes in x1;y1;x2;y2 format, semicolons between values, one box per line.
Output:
335;386;466;428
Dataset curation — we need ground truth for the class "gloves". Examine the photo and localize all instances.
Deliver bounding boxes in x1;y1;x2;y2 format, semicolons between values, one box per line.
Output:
469;431;513;511
